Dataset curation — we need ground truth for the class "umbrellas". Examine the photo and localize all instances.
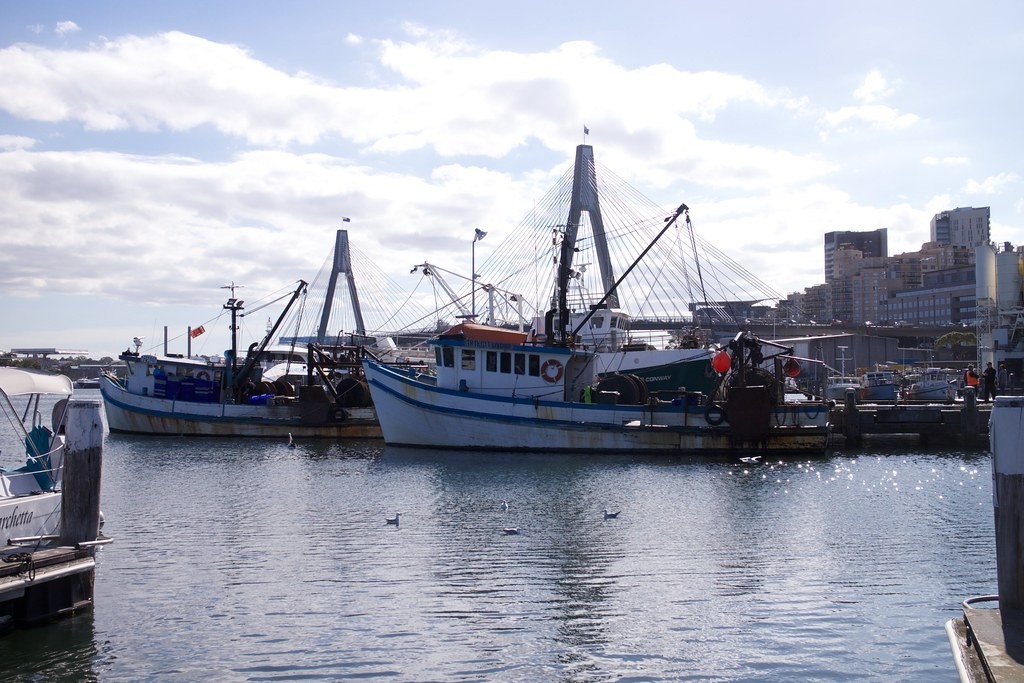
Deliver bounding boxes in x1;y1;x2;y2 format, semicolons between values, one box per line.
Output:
25;410;54;490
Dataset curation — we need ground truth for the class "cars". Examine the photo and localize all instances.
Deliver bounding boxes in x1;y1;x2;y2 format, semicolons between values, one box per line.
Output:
865;320;874;326
830;319;843;326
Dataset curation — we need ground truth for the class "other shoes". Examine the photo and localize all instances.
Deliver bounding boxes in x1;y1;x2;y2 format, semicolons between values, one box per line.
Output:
983;400;989;403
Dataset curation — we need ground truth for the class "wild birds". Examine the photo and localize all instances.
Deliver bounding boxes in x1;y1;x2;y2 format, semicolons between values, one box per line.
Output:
503;527;522;535
602;509;621;519
739;456;761;463
385;512;402;524
501;500;508;510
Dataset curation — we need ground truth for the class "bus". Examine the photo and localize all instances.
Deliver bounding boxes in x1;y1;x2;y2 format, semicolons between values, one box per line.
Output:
826;376;862;400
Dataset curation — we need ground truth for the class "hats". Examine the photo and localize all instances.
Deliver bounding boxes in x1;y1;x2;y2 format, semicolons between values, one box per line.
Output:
968;364;974;367
987;361;992;365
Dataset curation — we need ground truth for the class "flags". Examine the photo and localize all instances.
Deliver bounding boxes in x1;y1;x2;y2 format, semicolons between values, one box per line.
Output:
343;217;350;222
584;126;589;135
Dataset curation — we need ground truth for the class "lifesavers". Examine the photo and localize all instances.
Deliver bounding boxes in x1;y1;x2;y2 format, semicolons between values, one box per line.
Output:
704;405;725;426
196;371;211;381
541;359;563;383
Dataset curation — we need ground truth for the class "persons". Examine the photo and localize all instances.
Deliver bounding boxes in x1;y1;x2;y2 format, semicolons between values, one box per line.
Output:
967;364;979;396
999;364;1007;396
980;362;996;403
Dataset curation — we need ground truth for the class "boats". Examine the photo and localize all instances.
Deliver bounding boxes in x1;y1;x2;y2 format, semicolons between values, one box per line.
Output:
855;347;958;404
76;125;836;450
0;365;100;549
